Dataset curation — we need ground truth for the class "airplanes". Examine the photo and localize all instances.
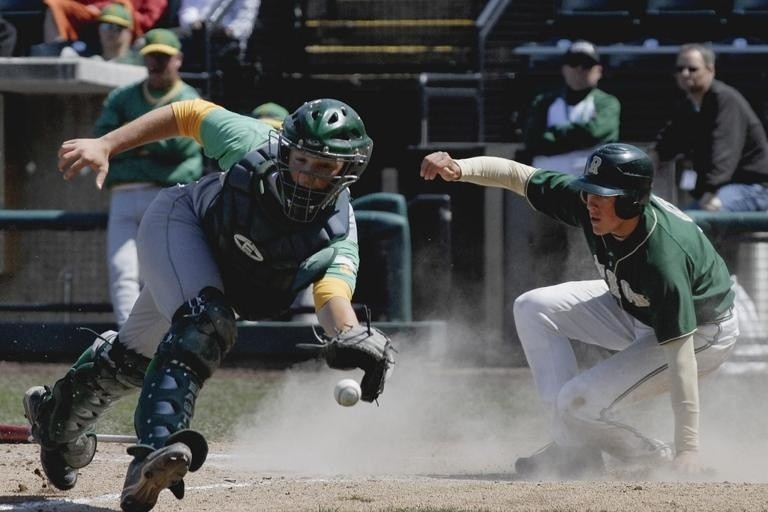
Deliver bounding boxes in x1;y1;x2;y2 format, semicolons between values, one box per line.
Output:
321;324;392;401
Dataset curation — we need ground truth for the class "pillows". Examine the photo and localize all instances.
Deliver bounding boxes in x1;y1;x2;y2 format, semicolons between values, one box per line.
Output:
266;99;373;223
571;142;654;219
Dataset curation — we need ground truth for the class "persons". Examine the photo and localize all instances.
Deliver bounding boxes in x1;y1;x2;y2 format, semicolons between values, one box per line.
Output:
173;0;259;67
524;38;621;180
234;102;318;324
23;98;394;512
92;28;203;328
33;1;166;61
647;42;768;213
83;2;147;66
420;144;741;480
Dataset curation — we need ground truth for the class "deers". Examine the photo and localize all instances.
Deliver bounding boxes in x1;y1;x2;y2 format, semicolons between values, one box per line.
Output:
563;65;600;91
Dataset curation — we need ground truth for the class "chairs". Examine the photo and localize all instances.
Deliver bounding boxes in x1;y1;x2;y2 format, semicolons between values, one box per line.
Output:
120;442;192;511
23;386;77;490
516;441;607;480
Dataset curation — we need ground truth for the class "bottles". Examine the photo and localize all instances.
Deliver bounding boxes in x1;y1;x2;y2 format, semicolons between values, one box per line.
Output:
252;103;289;129
98;3;131;28
567;40;600;64
139;29;181;58
